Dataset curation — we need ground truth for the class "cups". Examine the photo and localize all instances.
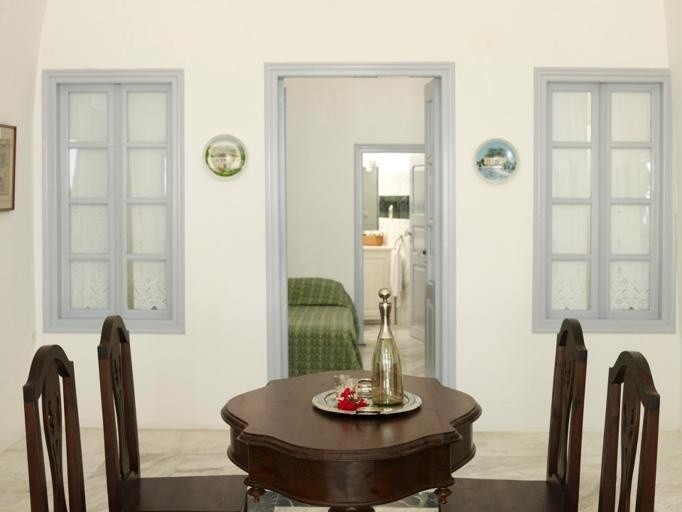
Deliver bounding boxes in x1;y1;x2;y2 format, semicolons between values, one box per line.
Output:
332;374;372;399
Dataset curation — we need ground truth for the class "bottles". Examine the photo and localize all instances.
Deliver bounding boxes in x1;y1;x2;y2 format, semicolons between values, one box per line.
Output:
371;289;403;407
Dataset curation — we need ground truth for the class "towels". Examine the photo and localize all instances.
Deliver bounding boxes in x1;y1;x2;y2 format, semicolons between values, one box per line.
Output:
389;249;405;306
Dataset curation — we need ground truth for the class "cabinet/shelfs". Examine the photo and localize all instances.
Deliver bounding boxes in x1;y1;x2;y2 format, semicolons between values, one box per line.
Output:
363;252;394;324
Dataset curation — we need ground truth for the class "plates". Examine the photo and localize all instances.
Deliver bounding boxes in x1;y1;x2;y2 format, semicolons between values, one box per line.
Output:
311;378;423;418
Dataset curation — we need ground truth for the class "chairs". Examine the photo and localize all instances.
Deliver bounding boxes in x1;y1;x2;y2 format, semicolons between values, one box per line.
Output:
434;319;588;510
596;350;662;510
96;315;247;510
19;343;87;510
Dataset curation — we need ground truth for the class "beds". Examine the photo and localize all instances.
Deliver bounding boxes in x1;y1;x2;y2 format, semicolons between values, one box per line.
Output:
288;277;359;377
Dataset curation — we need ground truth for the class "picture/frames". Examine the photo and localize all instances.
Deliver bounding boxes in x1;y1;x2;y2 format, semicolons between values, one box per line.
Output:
0;123;17;213
